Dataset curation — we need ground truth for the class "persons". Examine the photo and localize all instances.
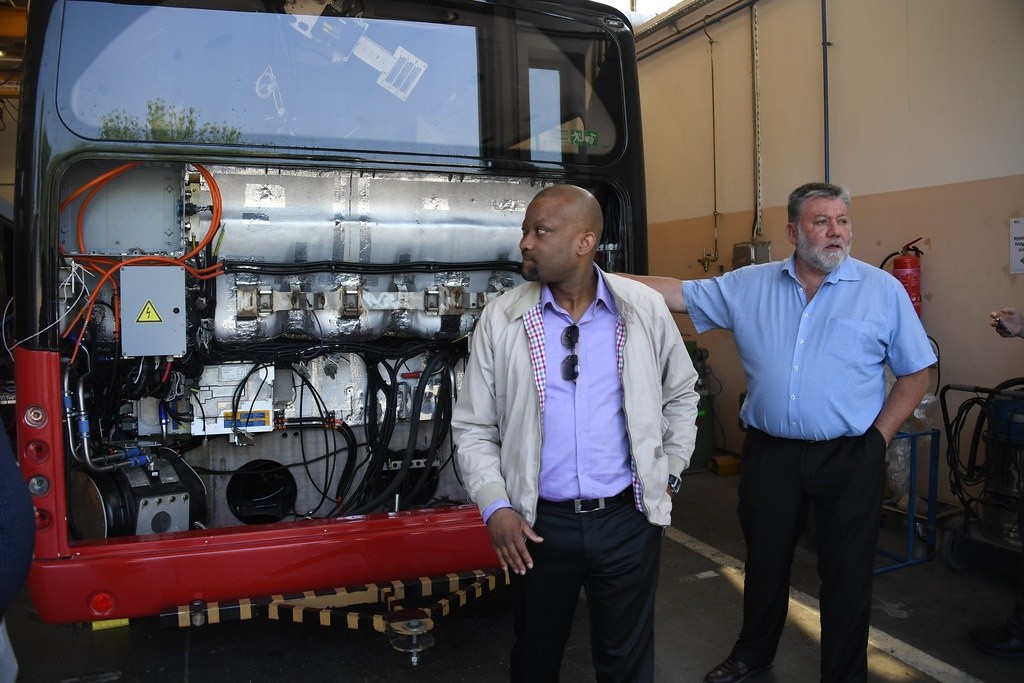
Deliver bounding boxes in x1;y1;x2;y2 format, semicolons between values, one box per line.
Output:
452;183;701;683
988;304;1024;339
604;182;940;683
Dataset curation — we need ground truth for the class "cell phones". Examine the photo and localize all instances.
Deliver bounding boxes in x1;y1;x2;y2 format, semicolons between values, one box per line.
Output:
994;318;1009;337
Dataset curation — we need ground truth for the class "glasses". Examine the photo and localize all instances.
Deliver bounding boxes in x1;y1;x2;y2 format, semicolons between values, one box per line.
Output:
560;322;580;384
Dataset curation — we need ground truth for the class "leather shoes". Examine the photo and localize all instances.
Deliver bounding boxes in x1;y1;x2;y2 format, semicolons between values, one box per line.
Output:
703;653;774;683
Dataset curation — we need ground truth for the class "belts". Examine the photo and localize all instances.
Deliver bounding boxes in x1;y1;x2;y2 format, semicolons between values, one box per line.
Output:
535;490;632;516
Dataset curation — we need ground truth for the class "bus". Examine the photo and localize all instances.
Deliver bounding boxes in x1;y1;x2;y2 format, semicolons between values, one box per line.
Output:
9;0;648;647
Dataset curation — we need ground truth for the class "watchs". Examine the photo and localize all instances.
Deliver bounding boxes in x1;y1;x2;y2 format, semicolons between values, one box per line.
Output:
669;474;681;494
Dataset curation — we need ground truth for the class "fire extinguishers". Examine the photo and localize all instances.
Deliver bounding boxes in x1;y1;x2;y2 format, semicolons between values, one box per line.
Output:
879;236;925;317
